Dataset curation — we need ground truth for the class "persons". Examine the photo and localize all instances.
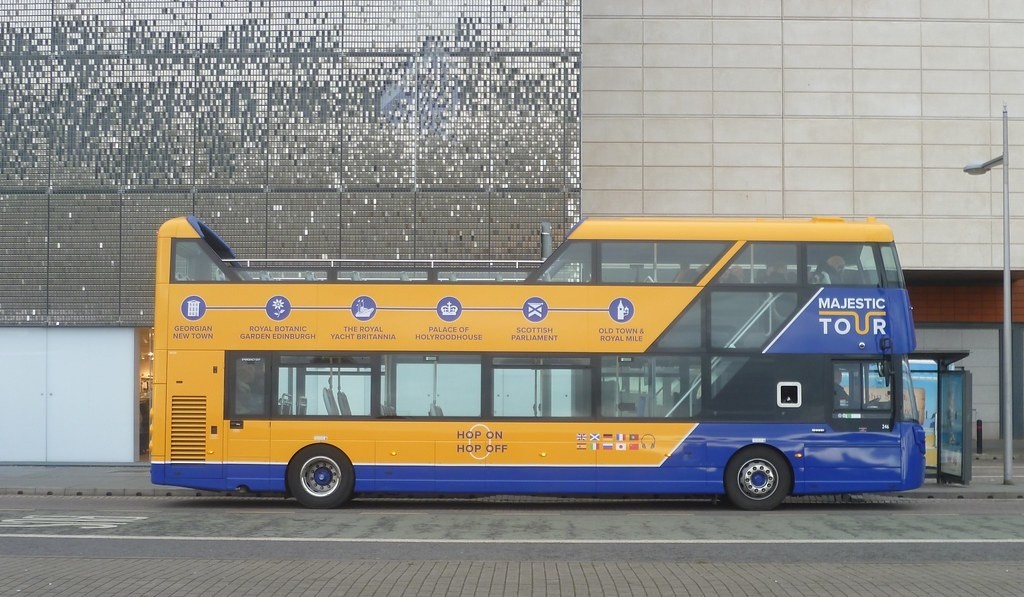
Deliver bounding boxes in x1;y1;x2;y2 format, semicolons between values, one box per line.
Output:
834;368;869;408
767;255;796;284
811;255;847;285
672;263;745;283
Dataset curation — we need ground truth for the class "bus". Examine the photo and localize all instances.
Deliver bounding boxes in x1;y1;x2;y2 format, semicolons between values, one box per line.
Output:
150;215;927;510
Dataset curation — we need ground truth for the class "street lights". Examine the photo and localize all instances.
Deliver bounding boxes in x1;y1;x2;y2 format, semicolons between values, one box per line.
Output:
963;103;1013;485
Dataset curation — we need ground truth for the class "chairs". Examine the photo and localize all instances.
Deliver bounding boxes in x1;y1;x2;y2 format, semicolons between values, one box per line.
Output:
428;402;442;416
382;402;396;416
280;393;308;416
323;389;351;416
